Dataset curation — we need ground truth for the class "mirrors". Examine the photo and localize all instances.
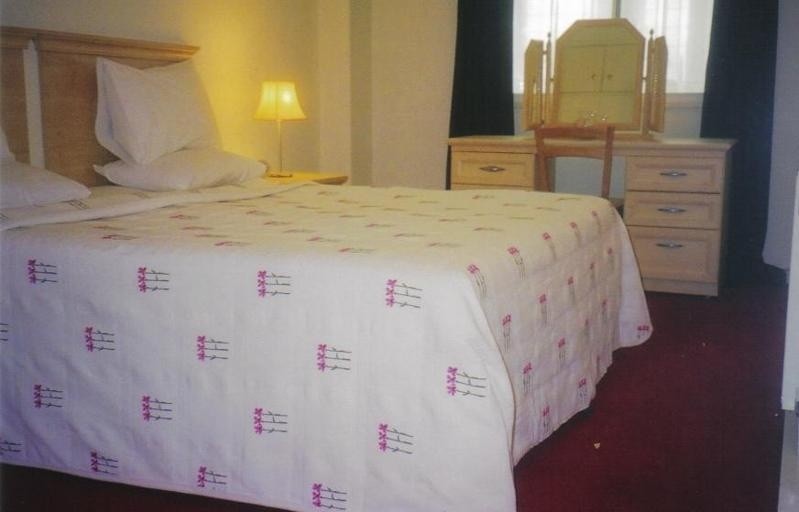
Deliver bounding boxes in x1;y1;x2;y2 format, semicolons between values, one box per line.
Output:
542;17;657;130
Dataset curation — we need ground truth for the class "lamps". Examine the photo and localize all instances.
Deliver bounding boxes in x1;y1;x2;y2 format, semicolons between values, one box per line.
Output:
249;76;310;182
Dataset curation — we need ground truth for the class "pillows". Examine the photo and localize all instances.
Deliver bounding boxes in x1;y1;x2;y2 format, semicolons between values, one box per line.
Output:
1;150;87;217
90;141;264;194
91;49;229;161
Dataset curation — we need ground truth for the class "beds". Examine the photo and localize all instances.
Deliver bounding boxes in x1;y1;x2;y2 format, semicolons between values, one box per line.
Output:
1;26;657;508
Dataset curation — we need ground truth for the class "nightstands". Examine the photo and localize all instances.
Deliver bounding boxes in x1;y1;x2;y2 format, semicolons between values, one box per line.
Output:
278;161;345;187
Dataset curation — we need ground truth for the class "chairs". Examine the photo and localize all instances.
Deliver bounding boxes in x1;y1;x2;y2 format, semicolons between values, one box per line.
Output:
526;118;627;213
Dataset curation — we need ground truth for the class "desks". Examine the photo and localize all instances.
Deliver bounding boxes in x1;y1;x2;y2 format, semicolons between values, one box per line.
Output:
445;123;738;298
